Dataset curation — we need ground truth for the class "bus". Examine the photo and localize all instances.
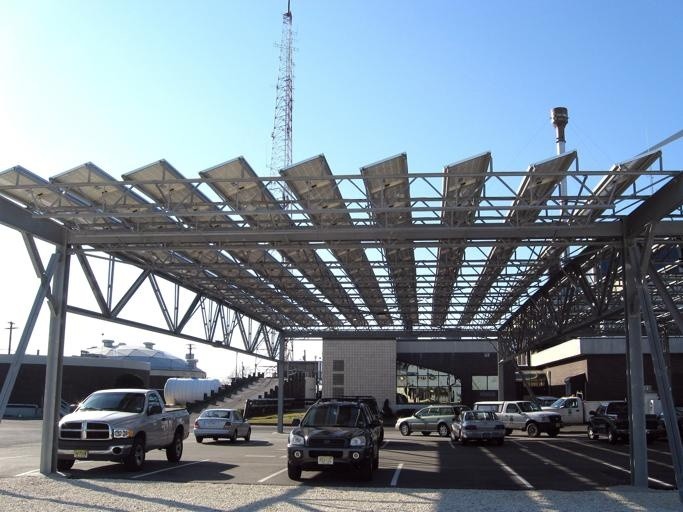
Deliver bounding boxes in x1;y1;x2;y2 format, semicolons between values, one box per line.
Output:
5;403;39;417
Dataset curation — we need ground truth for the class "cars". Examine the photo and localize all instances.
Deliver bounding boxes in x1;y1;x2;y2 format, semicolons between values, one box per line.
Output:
395;396;683;446
287;395;384;479
193;408;251;443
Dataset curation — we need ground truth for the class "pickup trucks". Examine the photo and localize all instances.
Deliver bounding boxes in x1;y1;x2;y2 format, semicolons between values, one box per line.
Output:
57;387;189;472
